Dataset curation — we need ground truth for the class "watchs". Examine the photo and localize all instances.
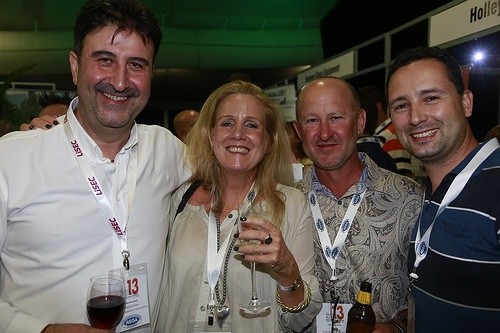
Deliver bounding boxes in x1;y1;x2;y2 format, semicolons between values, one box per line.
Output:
276;276;303;291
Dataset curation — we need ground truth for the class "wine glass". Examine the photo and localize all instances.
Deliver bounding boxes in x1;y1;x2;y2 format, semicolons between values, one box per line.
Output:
238;202;271;314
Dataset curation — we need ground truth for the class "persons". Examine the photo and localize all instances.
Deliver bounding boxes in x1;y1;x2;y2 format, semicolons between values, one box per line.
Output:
20;81;322;333
386;48;500;333
0;0;198;333
291;77;425;333
357;103;412;178
173;110;312;178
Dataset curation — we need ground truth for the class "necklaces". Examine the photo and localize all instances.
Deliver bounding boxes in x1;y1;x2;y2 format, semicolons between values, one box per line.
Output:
214;195;238;316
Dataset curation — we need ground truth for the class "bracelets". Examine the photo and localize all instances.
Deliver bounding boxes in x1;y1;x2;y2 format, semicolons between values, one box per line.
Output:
277;282;310;312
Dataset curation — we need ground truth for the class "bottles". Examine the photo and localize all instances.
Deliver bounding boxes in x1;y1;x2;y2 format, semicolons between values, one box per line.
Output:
346;282;376;333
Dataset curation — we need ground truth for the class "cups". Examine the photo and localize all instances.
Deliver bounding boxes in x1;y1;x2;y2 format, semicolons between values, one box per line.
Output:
86;275;126;330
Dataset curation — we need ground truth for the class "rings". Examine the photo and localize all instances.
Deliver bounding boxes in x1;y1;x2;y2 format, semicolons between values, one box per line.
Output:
264;234;273;245
